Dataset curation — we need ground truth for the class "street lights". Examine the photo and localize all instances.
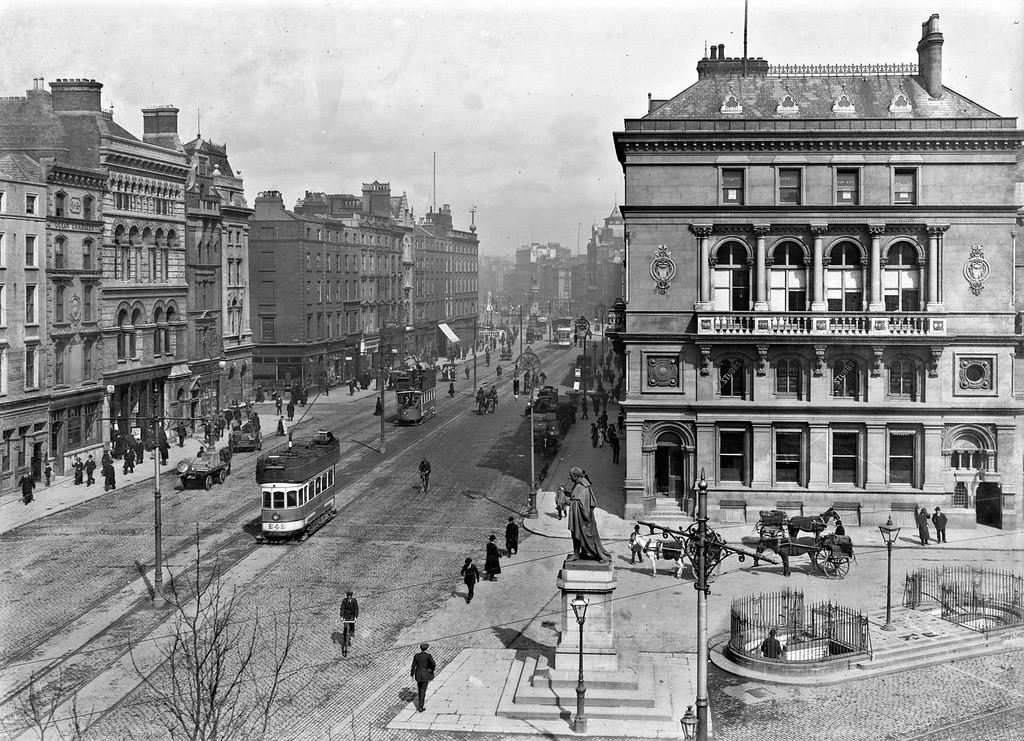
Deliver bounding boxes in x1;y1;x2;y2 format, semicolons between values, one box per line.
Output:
512;352;541;519
594;303;606;354
570;592;590;734
877;514;901;631
573;316;590;397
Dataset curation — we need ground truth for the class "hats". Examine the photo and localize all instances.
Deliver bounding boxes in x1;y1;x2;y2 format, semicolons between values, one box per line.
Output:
508;517;514;521
489;535;496;541
465;557;472;563
935;506;941;510
634;525;640;529
420;644;429;650
921;508;926;512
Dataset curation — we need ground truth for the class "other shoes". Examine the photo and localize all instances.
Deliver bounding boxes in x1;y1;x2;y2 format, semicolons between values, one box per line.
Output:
489;576;498;581
467;599;470;604
641;558;644;562
418;708;425;713
937;539;947;543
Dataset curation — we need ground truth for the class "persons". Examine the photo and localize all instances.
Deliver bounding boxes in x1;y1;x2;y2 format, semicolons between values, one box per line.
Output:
410;643;436;713
495;364;502;378
916;506;948;546
761;628;781;660
17;471;35;504
71;380;308;492
484;534;504;582
348;360;425;417
485;352;491;367
325;431;336;445
339;591;359;641
627;524;643;565
554;470;594;521
43;462;53;487
565;466;612;564
471;319;520;355
324;383;330;396
581;348;626;465
477;386;485;398
594;322;601;333
523;368;539;387
464;365;471;381
505;516;519;559
421;342;469;398
418;456;432;491
460;557;479;605
489;386;498;401
834;520;845;535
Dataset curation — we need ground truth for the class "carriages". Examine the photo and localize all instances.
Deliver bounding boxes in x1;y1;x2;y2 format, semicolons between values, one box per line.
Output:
755;506;841;540
754;533;855;579
526;331;536;344
500;351;514;359
479;392;497;414
629;530;723;583
177;417;262;489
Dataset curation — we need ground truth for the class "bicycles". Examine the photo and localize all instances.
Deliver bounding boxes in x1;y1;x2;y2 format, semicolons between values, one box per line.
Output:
420;470;429;492
341;614;355;658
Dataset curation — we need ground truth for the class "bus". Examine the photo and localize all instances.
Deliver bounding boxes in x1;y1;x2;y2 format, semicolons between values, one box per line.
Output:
556;326;574;349
256;432;340;544
396;369;437;426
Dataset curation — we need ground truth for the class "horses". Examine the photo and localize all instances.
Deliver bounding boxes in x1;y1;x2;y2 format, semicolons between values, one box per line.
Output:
477;393;490;415
787;508;841;539
627;533;686;579
538;432;551;459
756;536;822;578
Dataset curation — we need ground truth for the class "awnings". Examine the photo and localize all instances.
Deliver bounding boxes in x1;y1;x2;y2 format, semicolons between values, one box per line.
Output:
437;323;461;344
